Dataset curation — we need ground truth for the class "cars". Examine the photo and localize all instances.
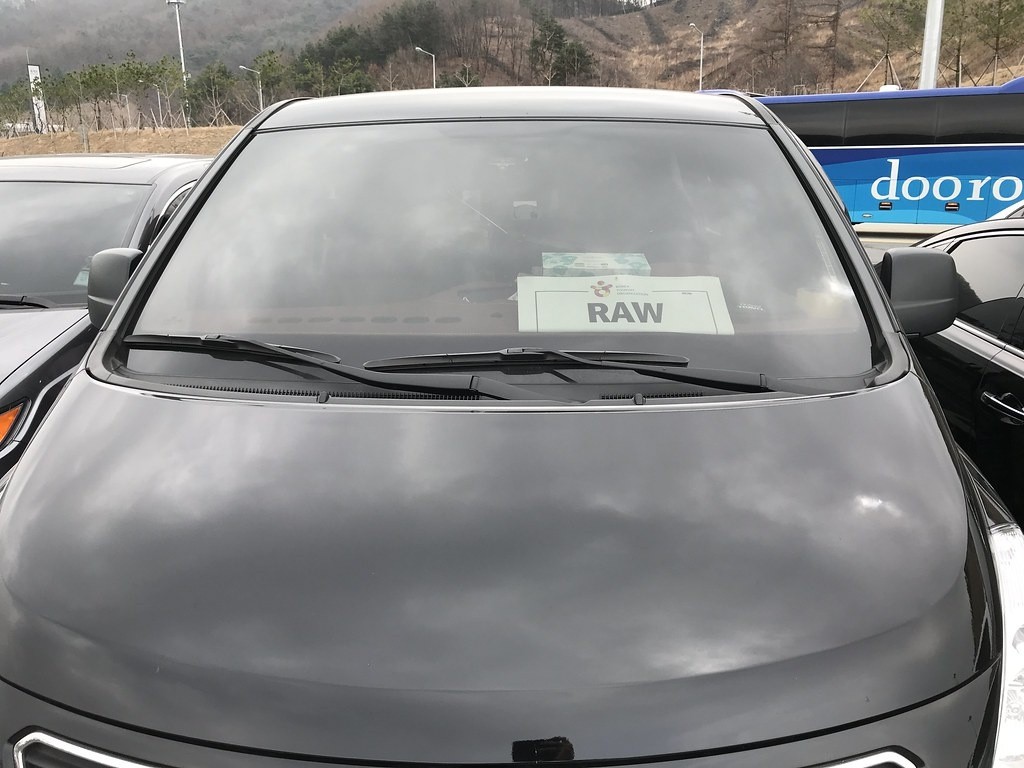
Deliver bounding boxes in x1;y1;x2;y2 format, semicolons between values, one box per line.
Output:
0;154;216;478
1;85;1024;768
827;216;1023;543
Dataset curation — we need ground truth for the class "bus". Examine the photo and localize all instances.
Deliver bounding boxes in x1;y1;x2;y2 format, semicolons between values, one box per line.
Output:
696;76;1024;247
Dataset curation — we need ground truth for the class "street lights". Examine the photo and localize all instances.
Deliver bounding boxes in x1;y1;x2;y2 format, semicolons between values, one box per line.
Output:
416;46;436;89
110;92;131;125
166;0;192;128
139;80;163;126
239;66;262;112
690;22;703;90
